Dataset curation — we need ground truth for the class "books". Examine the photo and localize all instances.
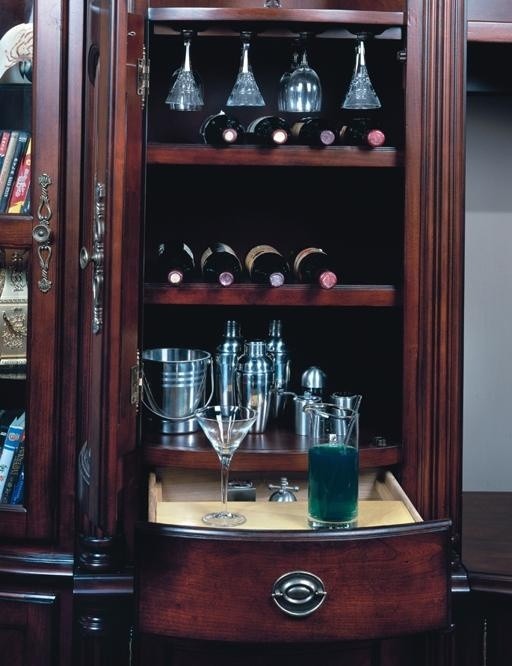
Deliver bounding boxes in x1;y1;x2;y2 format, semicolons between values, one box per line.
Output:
0;406;26;507
0;129;32;216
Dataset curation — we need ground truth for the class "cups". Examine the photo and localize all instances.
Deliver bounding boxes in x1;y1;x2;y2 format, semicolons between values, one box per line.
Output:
303;403;362;531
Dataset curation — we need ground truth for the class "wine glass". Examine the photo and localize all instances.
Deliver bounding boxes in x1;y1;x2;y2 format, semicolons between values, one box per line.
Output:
167;61;205;112
165;41;206;106
277;51;300;112
342;31;382;110
225;43;267;108
194;405;255;528
288;51;322;112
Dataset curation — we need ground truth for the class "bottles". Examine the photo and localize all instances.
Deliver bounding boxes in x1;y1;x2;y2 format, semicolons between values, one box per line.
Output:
199;239;242;286
244;242;291;287
200;109;246;152
289;245;337;290
236;339;276;434
290;115;336;145
292;390;321;436
301;366;329;403
156;239;195;287
338;116;385;147
265;317;292;418
331;387;358;436
215;319;246;417
247;115;289;144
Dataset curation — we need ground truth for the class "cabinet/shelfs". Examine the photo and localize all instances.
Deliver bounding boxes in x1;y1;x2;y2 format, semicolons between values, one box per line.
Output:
83;0;467;666
0;0;83;666
